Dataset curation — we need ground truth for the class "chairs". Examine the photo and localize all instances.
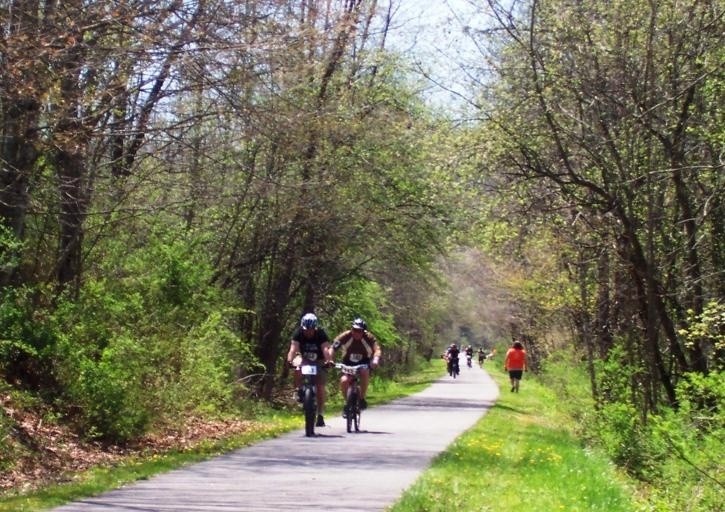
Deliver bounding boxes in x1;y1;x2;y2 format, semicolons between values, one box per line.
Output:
316;415;324;426
360;399;367;408
297;390;304;402
511;387;514;392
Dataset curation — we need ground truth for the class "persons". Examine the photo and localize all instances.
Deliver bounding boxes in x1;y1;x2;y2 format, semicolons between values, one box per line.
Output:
445;343;474;373
503;340;528;393
478;347;485;368
287;312;330;427
328;317;382;417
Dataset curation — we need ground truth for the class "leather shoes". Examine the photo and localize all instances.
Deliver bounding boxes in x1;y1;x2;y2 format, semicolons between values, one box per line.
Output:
287;361;292;367
372;360;378;367
324;360;335;367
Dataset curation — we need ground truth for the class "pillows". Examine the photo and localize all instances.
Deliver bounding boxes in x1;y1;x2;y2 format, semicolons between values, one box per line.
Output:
301;313;317;330
450;344;457;348
352;319;366;330
